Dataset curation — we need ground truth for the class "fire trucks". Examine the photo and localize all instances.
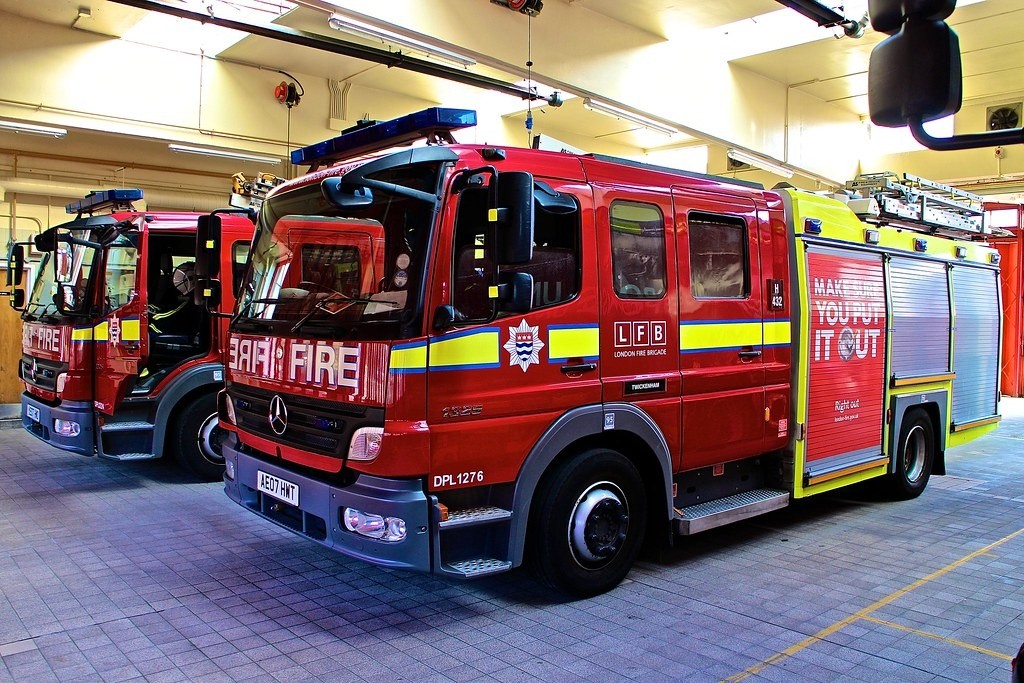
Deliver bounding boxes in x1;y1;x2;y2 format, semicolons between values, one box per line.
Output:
6;188;382;483
193;109;1005;604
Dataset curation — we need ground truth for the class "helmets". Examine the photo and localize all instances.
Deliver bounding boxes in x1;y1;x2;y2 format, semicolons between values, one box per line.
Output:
173;262;197;295
